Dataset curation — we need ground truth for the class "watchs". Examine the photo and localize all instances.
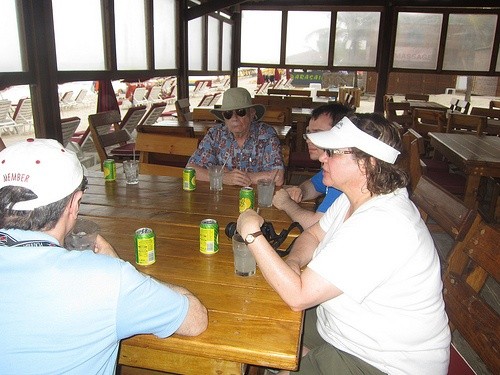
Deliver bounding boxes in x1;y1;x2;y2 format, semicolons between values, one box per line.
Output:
245;231;262;245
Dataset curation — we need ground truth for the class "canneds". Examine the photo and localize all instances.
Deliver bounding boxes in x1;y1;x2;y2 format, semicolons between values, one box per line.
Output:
199;219;219;255
239;187;255;213
183;191;194;210
182;168;196;191
103;159;116;181
134;228;156;266
104;180;117;200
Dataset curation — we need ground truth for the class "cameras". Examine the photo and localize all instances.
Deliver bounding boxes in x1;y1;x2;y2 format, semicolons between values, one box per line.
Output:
225;222;272;243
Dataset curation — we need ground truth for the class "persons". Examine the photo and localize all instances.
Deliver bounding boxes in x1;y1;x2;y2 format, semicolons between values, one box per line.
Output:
186;87;285;187
272;103;350;230
236;112;451;375
0;138;208;375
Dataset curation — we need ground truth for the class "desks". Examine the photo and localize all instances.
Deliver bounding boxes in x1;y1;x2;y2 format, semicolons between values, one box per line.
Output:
135;119;292;184
68;172;316;375
280;104;321;169
402;100;449;129
428;132;500;208
310;97;330;109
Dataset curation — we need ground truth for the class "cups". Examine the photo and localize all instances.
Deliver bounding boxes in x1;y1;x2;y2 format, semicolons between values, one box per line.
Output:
257;178;275;207
123;160;140;184
208;166;225;192
64;218;99;253
232;233;257;277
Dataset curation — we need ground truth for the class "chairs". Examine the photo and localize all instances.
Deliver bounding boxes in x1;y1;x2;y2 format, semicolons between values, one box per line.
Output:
0;77;338;178
384;94;500;375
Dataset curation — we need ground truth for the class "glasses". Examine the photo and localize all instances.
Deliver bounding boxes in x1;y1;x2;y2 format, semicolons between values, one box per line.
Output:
222;108;247;119
323;148;364;157
302;132;314;140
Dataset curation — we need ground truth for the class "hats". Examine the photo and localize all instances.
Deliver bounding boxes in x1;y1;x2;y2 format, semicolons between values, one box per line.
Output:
306;116;401;164
210;87;265;121
0;138;89;211
